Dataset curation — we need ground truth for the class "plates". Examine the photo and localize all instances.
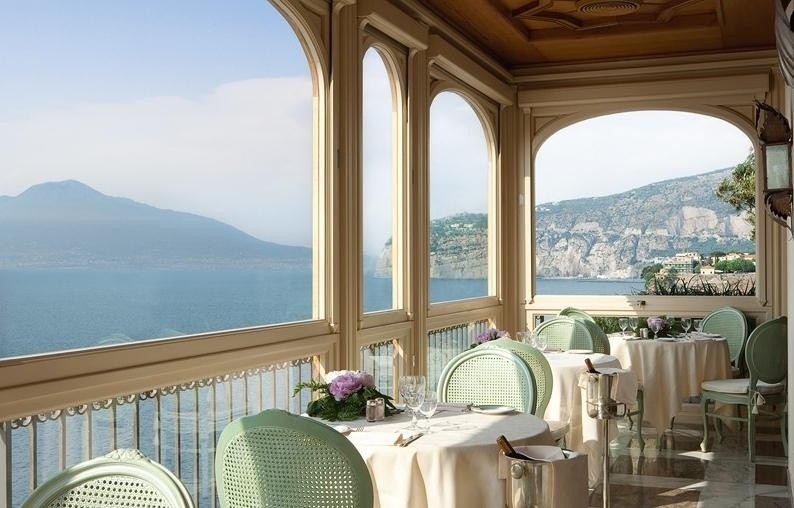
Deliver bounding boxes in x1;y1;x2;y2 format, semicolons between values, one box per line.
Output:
326;423;351;434
625;333;721;342
471;406;514;414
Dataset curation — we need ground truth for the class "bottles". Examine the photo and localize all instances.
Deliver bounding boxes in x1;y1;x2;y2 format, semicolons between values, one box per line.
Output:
585;358;601;374
496;434;533;461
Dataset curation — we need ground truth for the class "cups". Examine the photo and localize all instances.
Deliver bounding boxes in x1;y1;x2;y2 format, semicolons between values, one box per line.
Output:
366;400;377;422
375;398;385;421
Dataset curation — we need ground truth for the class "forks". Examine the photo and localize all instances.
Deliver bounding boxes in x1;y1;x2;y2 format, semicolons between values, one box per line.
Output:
351;425;365;432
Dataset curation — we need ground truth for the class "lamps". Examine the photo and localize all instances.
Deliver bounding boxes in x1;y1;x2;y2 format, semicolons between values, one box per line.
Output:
751;98;793;238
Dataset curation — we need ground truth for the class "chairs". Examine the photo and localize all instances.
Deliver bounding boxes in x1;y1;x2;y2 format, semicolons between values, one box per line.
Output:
18;448;194;508
213;409;373;508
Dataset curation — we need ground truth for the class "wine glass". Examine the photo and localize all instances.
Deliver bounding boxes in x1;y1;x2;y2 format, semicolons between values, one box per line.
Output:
398;376;438;432
515;332;548;355
618;316;704;340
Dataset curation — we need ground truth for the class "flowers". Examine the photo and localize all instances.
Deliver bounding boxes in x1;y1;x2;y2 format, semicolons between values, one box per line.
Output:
293;368;404;422
